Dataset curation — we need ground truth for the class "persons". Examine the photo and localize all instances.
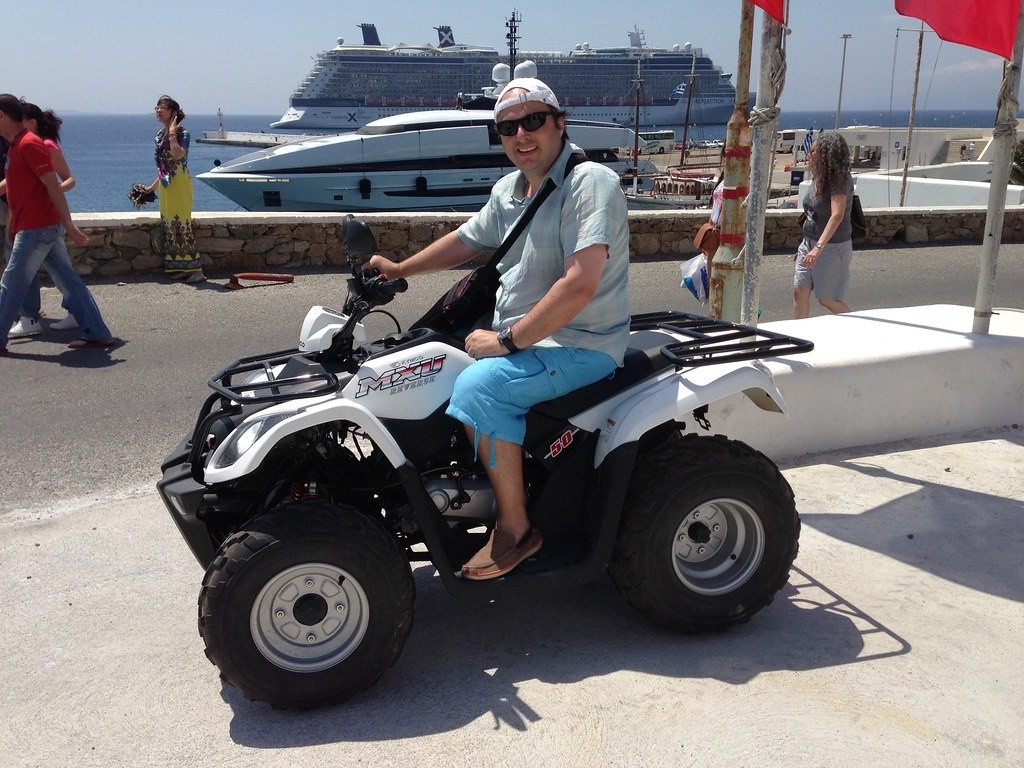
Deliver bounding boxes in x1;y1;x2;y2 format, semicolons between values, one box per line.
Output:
793;131;854;318
361;78;630;580
144;98;205;283
0;94;112;355
707;139;726;287
960;141;975;162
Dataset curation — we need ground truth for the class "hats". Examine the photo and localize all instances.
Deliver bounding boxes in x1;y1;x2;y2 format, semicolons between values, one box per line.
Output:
493;77;560;124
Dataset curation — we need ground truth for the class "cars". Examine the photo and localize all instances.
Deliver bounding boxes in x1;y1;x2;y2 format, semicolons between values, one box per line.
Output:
675;140;726;149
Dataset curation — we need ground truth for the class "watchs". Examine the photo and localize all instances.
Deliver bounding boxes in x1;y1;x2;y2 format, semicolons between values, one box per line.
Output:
497;326;518;352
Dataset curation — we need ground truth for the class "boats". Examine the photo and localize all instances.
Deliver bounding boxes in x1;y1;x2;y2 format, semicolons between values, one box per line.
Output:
193;106;662;214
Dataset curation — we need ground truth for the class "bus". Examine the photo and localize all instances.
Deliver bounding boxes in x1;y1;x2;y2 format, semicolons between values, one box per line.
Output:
621;130;675;155
771;129;823;154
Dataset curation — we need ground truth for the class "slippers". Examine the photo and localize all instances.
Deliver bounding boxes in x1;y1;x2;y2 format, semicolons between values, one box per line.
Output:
186;275;207;284
170;270;191;279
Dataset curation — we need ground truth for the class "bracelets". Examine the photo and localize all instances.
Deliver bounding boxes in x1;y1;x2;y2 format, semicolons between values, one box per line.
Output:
168;133;176;135
816;242;824;249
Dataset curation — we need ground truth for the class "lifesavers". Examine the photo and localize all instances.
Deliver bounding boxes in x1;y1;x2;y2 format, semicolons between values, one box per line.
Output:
566;110;571;116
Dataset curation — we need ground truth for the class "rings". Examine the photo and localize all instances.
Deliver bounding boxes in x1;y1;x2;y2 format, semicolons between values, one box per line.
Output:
810;258;812;262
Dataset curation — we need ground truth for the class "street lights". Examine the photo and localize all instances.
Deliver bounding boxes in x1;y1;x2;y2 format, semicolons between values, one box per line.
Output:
834;32;854;130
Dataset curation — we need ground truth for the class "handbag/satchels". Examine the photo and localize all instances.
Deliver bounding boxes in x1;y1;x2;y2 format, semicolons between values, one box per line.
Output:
692;223;720;256
679;253;709;308
408;266;500;336
796;194;867;241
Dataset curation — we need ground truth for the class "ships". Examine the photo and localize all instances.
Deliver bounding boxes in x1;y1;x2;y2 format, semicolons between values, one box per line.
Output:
197;19;760;149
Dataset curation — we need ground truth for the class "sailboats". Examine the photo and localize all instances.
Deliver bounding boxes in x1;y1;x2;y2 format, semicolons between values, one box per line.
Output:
585;56;719;210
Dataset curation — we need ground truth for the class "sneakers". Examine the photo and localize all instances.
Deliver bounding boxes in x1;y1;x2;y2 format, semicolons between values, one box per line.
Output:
460;520;543;581
49;313;80;329
8;315;42;335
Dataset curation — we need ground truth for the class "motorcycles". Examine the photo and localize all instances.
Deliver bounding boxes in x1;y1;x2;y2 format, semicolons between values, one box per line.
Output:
155;213;814;714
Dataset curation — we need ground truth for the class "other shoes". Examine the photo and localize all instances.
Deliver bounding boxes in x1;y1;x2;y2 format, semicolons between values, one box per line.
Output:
67;335;118;348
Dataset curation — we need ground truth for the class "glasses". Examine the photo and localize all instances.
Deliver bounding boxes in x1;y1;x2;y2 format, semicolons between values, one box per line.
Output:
154;106;169;111
495;111;554;137
